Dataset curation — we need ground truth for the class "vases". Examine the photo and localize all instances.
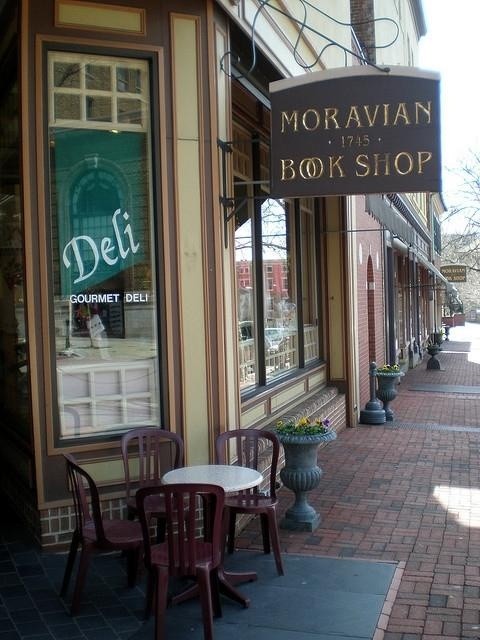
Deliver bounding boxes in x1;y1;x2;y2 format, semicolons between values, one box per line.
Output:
278;430;338;532
376;371;406;422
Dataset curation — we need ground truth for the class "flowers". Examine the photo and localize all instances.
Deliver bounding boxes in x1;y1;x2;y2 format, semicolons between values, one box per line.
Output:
277;416;330;435
377;363;401;373
